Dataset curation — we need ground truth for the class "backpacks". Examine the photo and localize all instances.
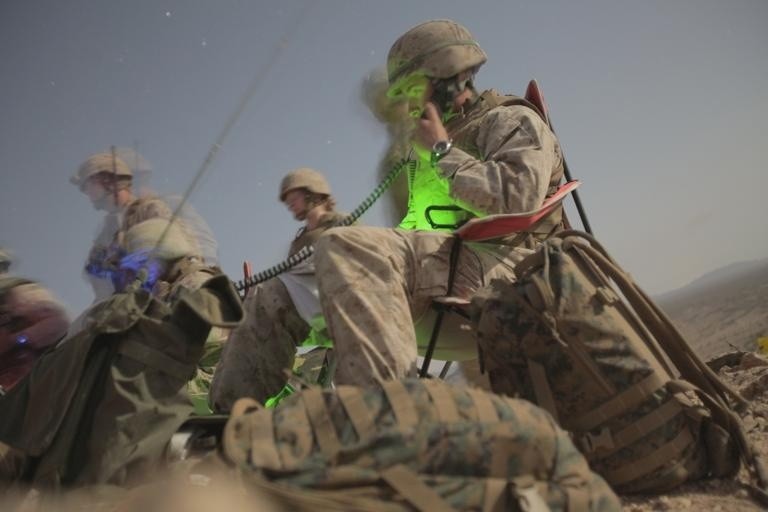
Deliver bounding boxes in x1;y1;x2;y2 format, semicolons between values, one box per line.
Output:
477;228;767;505
217;377;624;512
0;273;245;496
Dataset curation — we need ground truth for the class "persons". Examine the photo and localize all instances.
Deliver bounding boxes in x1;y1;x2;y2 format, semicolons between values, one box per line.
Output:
179;19;571;462
2;246;70;389
273;169;358;265
69;144;205;402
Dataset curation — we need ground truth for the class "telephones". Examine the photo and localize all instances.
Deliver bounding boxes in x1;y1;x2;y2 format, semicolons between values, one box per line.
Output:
421;77;456;119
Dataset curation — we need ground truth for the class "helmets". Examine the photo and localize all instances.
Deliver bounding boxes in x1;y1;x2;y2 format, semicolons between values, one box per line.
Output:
122;217;194;264
77;152;133;195
279;169;331;200
385;19;486;102
